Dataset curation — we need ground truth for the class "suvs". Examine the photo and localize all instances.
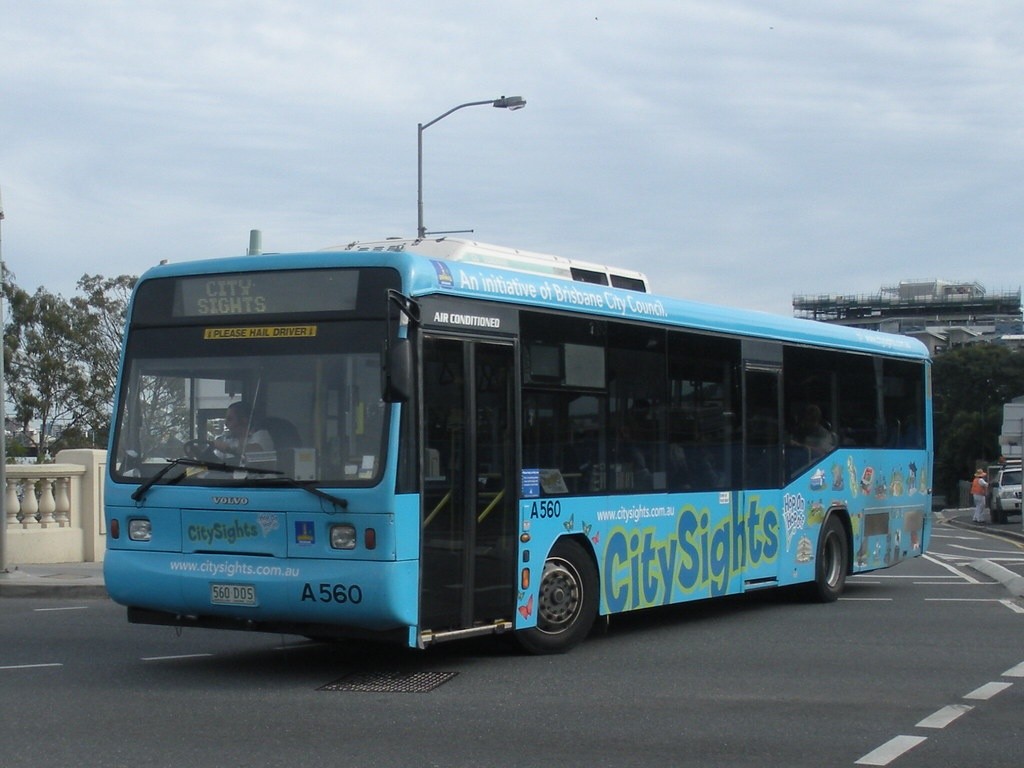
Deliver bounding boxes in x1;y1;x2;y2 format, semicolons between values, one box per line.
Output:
989;469;1023;525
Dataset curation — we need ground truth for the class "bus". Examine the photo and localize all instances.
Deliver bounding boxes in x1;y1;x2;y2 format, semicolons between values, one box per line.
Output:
100;239;934;659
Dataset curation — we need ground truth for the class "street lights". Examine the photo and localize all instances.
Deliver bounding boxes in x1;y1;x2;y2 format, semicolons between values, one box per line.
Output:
416;94;526;238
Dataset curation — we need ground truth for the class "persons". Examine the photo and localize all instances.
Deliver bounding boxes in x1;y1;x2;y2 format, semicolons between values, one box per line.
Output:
44;448;51;461
970;468;989;523
789;405;832;458
997;455;1006;465
212;402;276;479
